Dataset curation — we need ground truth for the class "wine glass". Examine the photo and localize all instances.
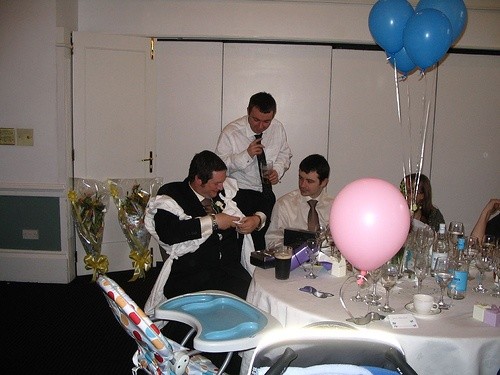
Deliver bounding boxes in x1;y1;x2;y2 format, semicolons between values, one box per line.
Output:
304;218;500;311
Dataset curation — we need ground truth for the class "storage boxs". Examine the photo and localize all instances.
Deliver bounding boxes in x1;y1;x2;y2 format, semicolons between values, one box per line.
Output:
284;228;318;249
250;250;275;270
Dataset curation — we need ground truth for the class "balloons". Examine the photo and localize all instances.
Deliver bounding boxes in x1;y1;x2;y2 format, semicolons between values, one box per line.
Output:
403;8;452;71
368;0;414;53
385;48;417;79
416;0;467;44
330;177;411;286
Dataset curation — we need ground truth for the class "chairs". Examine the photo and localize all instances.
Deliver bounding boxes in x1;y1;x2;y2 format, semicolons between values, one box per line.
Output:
96;274;284;375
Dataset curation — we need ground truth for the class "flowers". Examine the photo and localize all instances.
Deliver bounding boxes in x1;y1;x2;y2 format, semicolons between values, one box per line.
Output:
67;189;107;282
111;184;153;281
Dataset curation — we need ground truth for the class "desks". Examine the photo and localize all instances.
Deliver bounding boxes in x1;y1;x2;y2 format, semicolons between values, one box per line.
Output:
237;247;500;375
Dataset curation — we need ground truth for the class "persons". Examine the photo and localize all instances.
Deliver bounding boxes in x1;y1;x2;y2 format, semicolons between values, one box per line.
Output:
470;199;500;249
400;173;446;233
214;91;293;251
153;149;267;300
265;153;335;249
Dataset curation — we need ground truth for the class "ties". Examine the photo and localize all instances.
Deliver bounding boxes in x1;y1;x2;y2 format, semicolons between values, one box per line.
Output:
200;198;216;215
307;199;321;234
254;132;274;206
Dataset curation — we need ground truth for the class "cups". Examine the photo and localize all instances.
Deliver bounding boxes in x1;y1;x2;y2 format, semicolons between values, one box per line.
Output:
413;293;439;313
274;246;293;281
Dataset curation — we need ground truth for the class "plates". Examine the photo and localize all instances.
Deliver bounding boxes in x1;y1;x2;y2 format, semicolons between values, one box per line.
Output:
405;300;442;316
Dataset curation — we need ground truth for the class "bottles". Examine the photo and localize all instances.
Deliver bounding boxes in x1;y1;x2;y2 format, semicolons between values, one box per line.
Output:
430;223;449;277
259;161;273;184
446;236;468;300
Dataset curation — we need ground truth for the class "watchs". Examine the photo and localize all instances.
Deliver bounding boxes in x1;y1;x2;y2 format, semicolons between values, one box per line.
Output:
210;212;218;232
